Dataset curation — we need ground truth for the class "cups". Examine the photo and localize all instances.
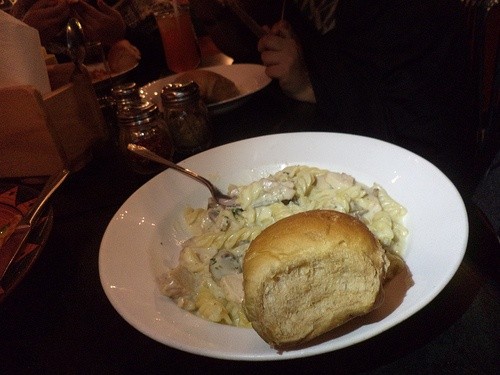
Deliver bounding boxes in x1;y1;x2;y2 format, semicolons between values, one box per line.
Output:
67;41;112;88
153;10;202;73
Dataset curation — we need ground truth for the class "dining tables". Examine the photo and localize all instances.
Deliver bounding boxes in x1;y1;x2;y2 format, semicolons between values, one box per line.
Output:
0;18;500;375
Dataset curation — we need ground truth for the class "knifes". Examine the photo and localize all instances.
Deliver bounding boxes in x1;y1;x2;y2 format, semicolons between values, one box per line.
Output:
0;162;71;281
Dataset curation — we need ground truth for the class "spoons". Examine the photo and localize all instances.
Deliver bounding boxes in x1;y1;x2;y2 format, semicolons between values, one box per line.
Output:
66;18;84;83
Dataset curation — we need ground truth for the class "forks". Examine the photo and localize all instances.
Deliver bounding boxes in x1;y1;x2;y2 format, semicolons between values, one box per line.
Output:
127;145;241;211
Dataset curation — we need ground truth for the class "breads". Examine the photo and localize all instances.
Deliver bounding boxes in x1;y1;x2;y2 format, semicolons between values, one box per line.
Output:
242;208;390;347
168;70;241;102
106;40;141;74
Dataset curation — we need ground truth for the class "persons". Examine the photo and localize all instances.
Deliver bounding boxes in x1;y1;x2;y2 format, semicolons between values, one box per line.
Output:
257;0;470;150
11;0;126;47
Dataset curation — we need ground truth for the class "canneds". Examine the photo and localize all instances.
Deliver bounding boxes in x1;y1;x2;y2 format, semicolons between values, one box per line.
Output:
112;80;212;182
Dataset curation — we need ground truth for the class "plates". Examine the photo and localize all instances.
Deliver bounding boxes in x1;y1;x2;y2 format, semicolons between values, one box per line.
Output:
0;183;53;303
98;132;469;360
45;63;138;89
137;64;273;115
